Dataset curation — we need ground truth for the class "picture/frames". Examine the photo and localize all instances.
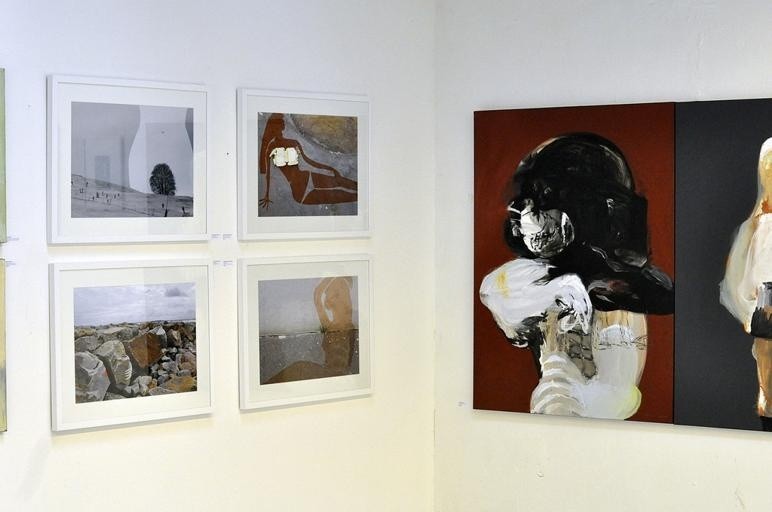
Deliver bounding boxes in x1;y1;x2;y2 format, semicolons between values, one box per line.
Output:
45;76;208;244
236;86;371;243
50;262;212;432
236;253;372;410
